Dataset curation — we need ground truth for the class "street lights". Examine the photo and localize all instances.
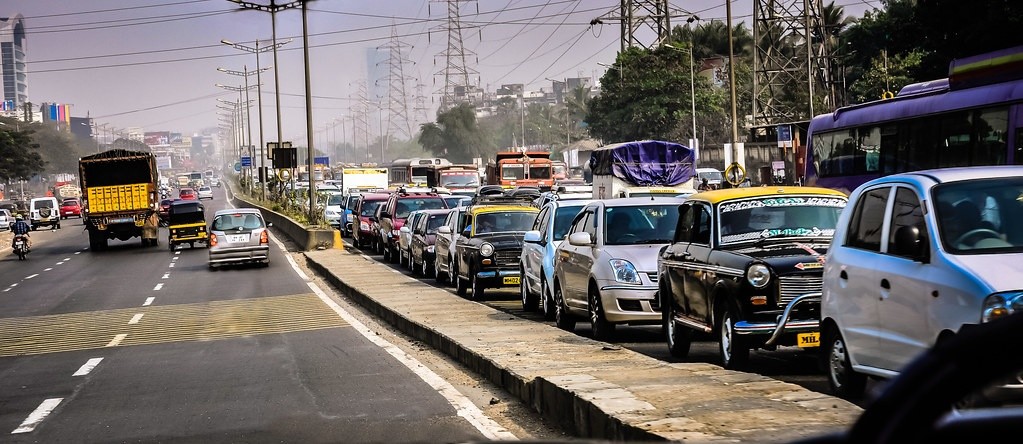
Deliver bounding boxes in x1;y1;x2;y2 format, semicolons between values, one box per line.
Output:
545;77;571;176
214;67;278;202
504;86;526;155
597;60;623;88
220;39;297;202
665;44;698;169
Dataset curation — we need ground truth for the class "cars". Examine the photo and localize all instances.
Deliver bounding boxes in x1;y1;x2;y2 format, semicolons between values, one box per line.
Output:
209;207;273;270
289;162;700;341
820;165;1023;407
0;209;16;230
161;168;221;201
0;181;84;220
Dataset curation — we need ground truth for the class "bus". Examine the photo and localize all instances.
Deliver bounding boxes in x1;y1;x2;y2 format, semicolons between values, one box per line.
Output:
803;47;1023;198
391;157;451;184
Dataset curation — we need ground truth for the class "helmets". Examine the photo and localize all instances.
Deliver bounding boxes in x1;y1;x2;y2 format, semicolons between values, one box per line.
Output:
15;214;24;220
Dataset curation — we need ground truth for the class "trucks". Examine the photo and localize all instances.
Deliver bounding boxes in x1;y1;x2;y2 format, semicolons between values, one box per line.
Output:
590;141;698;200
426;164;482;196
484;150;553;192
78;147;162;251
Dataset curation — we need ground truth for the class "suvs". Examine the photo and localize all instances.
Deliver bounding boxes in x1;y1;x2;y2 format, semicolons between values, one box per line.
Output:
31;197;61;230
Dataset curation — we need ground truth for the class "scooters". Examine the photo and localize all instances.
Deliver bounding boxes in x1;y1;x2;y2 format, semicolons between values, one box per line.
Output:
158;186;172;200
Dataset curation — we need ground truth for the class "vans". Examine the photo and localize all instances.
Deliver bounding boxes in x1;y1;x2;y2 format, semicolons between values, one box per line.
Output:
693;168;725;189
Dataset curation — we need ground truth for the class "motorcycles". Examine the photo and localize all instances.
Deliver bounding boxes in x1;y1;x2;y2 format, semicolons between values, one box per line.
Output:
167;201;209;252
9;225;34;262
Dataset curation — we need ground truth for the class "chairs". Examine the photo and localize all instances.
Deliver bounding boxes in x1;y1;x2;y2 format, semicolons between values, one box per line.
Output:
244;215;255;227
477;216;496;225
221;216;232;228
951;197;997;248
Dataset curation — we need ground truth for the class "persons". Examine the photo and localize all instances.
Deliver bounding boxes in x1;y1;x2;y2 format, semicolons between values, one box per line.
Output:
698;207;756;241
778;207;820;231
698;178;713;191
607;212;633;243
12;213;30;249
463;217;494;236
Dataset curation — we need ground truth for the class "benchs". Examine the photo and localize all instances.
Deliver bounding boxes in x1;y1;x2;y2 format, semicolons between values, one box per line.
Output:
819;155;866;175
